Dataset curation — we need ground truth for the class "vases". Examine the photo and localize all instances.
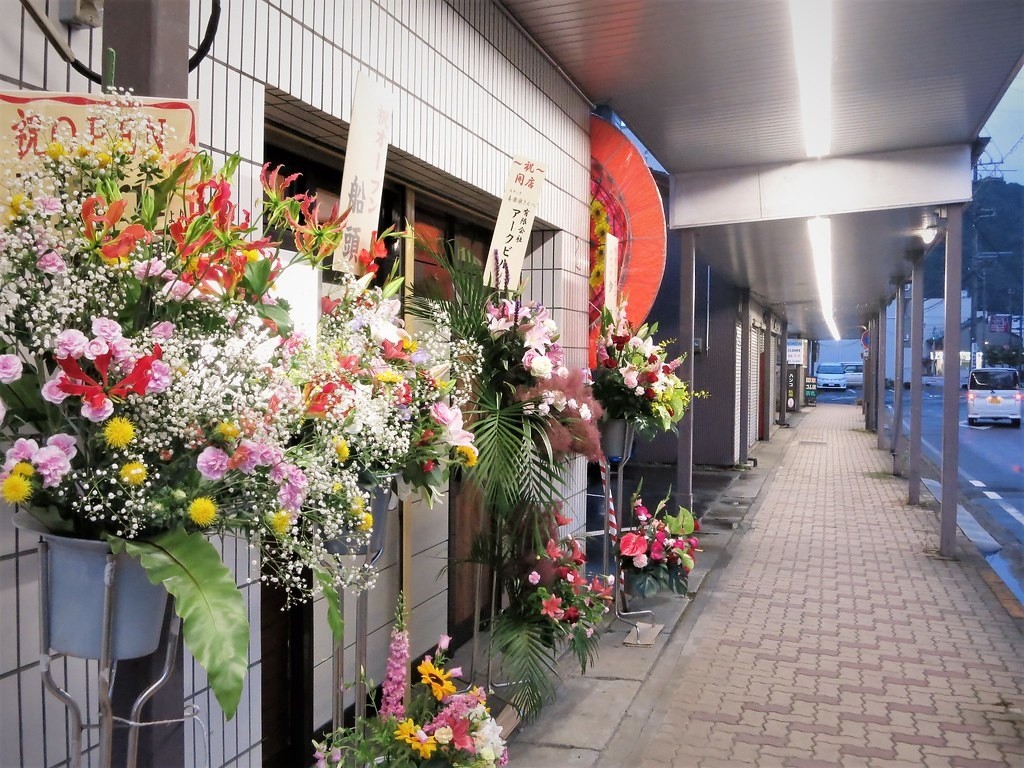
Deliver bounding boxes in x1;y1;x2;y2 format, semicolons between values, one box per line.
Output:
11;511;167;659
598;419;634;456
624;569;659;595
316;469;399;555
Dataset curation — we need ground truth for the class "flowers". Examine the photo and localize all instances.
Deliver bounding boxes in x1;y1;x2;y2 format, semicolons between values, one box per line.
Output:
0;87;707;767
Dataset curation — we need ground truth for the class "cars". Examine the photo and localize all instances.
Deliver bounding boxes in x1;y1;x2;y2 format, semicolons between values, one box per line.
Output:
815;364;847;390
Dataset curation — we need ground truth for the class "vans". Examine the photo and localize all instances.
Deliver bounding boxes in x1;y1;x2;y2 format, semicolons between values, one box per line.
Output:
962;368;1023;428
840;362;864;388
